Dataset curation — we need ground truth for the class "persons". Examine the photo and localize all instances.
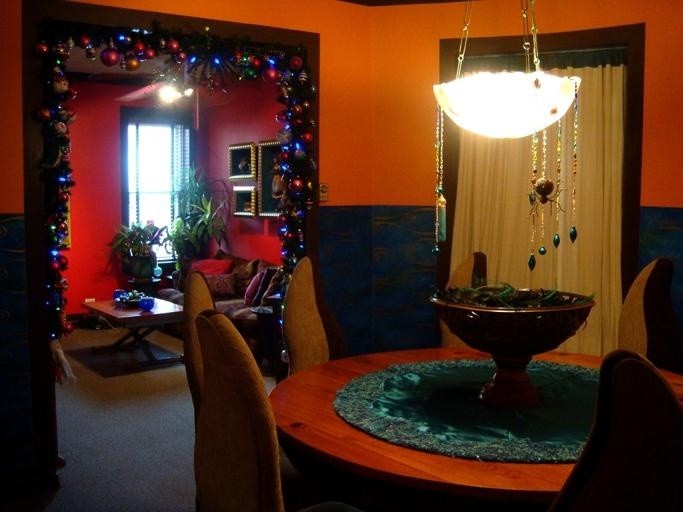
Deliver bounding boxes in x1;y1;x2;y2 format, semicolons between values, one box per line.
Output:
47;320;79;470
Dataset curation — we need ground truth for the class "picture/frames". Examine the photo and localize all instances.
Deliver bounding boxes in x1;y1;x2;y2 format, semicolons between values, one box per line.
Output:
226;141;284;218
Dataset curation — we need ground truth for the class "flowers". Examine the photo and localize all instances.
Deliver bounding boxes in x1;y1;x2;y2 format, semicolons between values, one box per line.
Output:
109;221;167;256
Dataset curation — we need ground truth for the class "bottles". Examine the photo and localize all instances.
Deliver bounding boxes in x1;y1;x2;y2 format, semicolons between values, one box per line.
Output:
113;288;127;303
138;296;155;313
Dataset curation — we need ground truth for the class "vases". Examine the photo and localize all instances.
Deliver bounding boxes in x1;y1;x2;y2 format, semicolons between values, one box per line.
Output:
123;256;158;278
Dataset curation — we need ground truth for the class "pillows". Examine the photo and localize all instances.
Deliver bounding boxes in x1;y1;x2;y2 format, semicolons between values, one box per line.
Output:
188;257;235;300
243;267;282;307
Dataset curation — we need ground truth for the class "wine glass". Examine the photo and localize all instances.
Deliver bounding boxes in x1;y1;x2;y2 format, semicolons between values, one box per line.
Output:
429;287;598;410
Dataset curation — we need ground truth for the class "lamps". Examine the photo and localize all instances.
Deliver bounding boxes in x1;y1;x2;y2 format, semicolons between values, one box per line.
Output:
432;1;581;142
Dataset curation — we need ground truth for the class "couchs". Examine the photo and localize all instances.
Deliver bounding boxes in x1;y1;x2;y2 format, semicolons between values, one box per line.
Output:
156;250;284;373
